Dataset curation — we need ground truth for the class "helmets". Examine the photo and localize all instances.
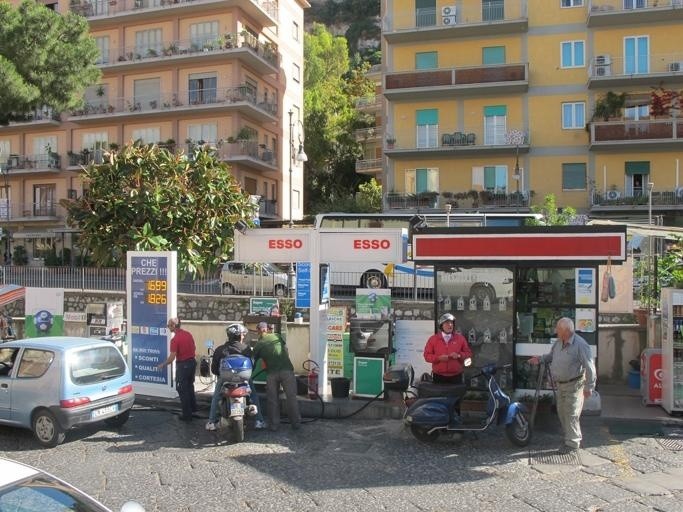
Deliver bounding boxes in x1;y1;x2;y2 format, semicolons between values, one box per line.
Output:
226;324;248;337
438;313;456;329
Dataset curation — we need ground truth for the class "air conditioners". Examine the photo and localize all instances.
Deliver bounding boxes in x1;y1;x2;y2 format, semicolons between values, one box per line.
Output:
588;54;612;77
668;61;683;72
6;159;18;168
605;189;621;201
676;188;683;198
441;5;457;26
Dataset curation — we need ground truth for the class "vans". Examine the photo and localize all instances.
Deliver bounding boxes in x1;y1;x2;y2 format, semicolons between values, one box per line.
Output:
219;258;287;297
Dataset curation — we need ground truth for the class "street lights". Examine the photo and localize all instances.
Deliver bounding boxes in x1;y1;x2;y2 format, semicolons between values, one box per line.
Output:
289;105;308;296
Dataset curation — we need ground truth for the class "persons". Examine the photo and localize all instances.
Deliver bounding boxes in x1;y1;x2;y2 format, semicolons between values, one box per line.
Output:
7;350;19;377
157;317;199;422
424;312;473;416
634;180;642;198
253;321;301;431
205;324;267;431
528;317;598;455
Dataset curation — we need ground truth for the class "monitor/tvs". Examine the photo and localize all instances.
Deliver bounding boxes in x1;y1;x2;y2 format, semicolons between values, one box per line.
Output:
348;320;391;357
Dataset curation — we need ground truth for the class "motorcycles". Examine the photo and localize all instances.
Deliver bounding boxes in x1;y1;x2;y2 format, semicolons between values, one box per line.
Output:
385;358;531;448
203;342;258;441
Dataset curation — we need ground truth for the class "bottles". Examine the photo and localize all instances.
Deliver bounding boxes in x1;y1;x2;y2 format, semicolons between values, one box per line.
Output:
673;305;683;343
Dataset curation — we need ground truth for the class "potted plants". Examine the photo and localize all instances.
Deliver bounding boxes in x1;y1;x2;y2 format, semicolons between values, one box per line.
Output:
385;189;534;209
633;255;664;327
591;193;683;206
43;248;70;274
10;245;28;275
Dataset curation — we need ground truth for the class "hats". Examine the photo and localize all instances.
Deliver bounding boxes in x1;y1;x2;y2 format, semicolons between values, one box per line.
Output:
257;322;267;328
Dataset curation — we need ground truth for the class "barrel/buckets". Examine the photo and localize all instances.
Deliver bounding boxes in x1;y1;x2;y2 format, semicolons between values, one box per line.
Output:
627;369;640;389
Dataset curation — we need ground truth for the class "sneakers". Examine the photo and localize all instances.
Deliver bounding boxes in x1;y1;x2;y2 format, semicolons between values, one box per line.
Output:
255;420;267;429
558;445;577;454
205;422;217;431
179;414;192;421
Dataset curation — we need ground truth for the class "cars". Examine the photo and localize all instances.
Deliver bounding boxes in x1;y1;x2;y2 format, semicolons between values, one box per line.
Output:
1;335;135;441
0;455;149;512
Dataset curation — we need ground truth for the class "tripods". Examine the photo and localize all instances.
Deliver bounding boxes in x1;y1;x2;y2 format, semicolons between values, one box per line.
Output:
530;363;555;431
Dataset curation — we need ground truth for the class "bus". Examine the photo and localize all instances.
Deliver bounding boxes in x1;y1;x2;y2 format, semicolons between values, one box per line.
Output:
582;218;683;290
312;211;550;301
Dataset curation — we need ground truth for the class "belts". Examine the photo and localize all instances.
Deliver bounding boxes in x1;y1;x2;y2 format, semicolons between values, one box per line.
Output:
559;376;583;384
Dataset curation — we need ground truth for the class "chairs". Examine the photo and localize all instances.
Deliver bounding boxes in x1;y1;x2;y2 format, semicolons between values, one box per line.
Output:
441;132;475;147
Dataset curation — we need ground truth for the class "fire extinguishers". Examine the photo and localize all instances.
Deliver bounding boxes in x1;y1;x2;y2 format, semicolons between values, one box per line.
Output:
308;366;318;399
